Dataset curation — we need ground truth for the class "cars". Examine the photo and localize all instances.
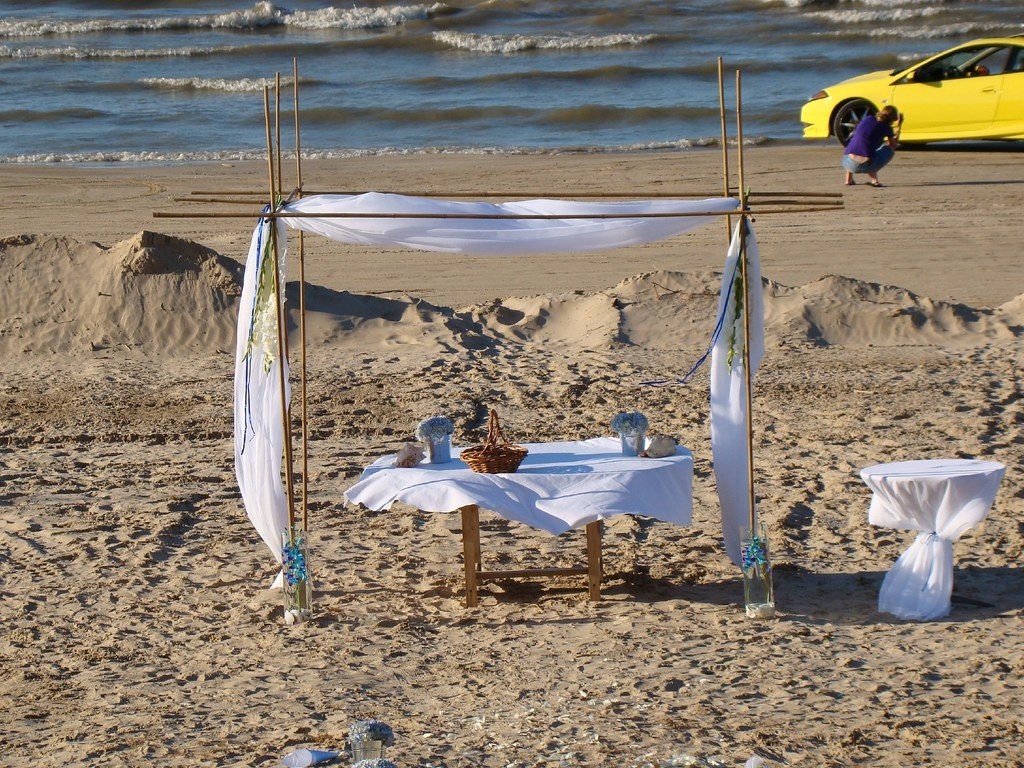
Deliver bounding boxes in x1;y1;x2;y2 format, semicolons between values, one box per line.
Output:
800;37;1024;148
944;33;1024;79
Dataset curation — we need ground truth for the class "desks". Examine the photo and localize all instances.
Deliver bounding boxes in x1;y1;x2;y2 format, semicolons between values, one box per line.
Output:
860;460;1005;621
342;438;692;611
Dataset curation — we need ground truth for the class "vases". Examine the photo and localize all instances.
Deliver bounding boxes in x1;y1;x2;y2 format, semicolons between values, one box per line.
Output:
745;564;775;620
283;582;302;623
428;434;451;464
621;433;646;456
352;740;386;761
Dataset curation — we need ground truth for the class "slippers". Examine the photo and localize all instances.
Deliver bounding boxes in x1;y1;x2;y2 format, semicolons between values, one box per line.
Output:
866;181;881;188
845;180;855;185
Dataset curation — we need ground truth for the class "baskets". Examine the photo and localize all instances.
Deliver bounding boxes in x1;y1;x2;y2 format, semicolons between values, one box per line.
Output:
461;409;528;473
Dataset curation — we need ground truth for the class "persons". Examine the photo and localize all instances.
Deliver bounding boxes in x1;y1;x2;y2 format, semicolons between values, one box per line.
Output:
841;105;904;187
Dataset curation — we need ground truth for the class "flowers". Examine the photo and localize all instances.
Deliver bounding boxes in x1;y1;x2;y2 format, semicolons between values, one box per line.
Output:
277;527;308;586
417;415;454;441
352;757;399;768
345;719;395;745
612;412;651;436
740;521;773;607
